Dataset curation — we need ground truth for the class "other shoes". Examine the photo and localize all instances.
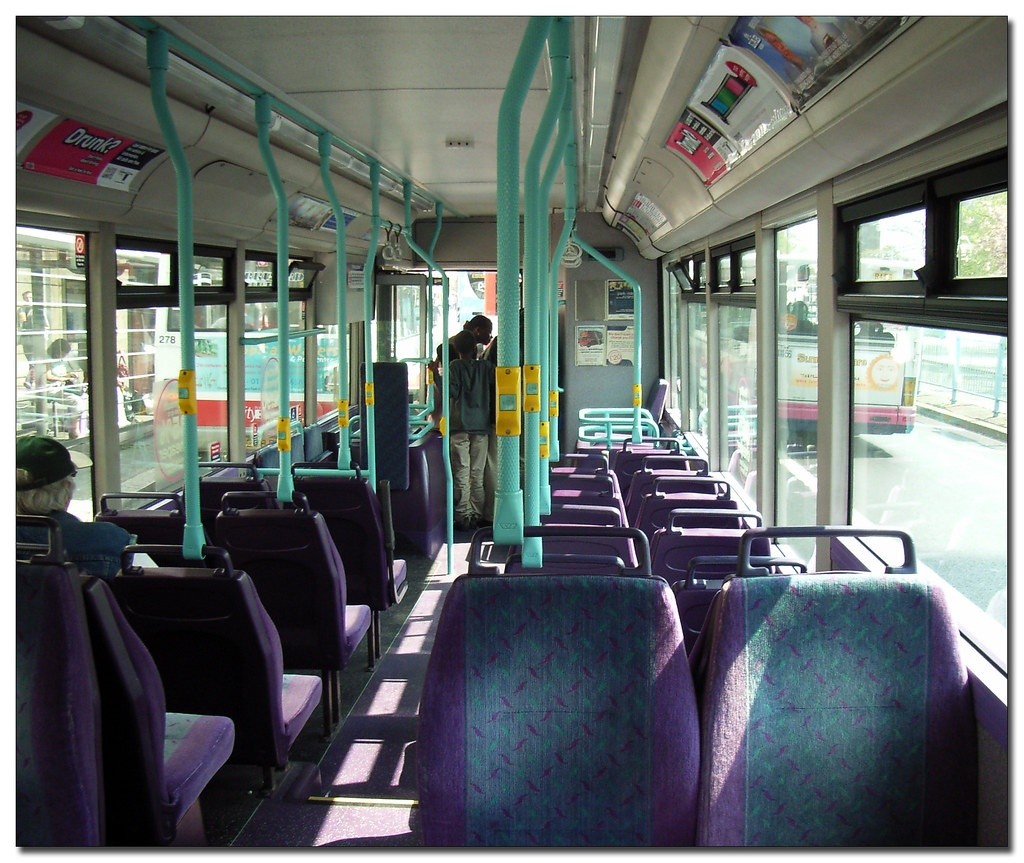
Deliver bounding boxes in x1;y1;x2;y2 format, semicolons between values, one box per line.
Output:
471;519;479;529
478;517;493;528
453;517;470;532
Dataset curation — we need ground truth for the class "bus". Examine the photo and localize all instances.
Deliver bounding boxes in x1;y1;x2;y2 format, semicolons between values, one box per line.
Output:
697;258;918;441
154;252;441;452
456;271;484;324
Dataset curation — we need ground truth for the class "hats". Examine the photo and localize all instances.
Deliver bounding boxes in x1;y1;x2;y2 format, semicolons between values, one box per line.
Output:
16;435;92;491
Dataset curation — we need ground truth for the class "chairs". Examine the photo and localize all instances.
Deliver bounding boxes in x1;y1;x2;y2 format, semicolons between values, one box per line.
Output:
15;461;409;849
420;380;982;847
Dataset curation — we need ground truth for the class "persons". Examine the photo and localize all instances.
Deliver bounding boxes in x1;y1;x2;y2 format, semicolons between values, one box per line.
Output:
45;339;89;439
758;27;819;88
430;309;526;531
17;435;158;581
16;291;49;388
17;110;32;129
797;16;851;66
